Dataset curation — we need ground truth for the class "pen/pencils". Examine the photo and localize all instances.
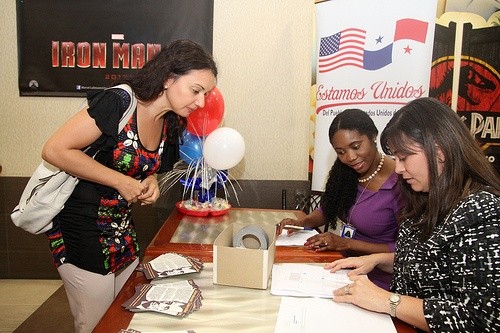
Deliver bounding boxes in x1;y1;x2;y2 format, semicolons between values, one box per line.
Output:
276;223;313;230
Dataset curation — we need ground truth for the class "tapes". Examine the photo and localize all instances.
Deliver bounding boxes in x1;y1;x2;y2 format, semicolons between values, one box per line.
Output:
234;224;268;250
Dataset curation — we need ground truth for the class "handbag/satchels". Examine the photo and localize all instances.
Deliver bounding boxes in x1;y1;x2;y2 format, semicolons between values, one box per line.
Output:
9;83;138;235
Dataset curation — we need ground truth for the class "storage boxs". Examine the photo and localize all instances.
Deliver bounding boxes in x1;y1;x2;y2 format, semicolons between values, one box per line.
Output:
213;221;275;291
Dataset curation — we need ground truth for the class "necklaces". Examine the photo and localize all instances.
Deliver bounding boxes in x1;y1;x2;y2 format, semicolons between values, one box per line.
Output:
358;151;384;182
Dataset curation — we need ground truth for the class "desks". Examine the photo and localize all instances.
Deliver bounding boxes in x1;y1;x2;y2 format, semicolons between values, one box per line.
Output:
91;207;417;333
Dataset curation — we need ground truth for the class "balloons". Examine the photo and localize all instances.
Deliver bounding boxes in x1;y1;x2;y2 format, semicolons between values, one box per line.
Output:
179;86;245;170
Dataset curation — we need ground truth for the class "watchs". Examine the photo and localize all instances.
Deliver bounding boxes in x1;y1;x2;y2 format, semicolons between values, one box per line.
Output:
389;294;401;317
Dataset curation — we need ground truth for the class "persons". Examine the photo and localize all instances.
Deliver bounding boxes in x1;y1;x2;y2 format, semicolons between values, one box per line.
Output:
344;231;350;237
276;108;400;291
42;39;217;333
324;97;500;333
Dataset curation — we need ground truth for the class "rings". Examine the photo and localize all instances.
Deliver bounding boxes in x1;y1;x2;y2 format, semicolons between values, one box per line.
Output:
325;241;327;246
344;287;350;295
